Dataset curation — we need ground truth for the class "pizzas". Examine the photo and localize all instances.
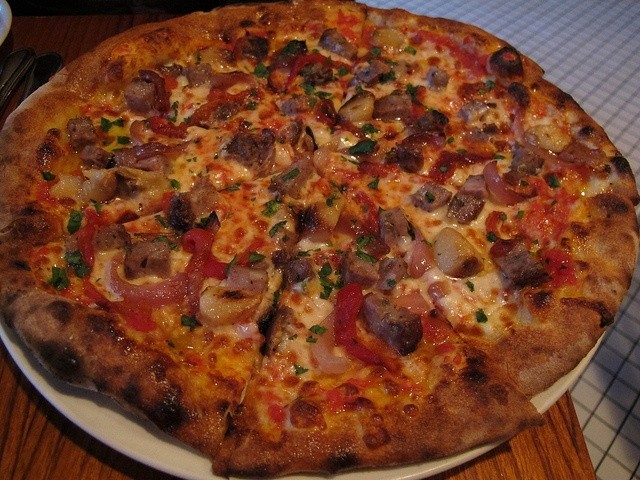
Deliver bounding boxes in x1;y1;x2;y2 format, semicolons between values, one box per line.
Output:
0;2;640;476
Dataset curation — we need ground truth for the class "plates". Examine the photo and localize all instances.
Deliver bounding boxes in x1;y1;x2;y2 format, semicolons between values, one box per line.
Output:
1;324;605;477
0;0;13;47
